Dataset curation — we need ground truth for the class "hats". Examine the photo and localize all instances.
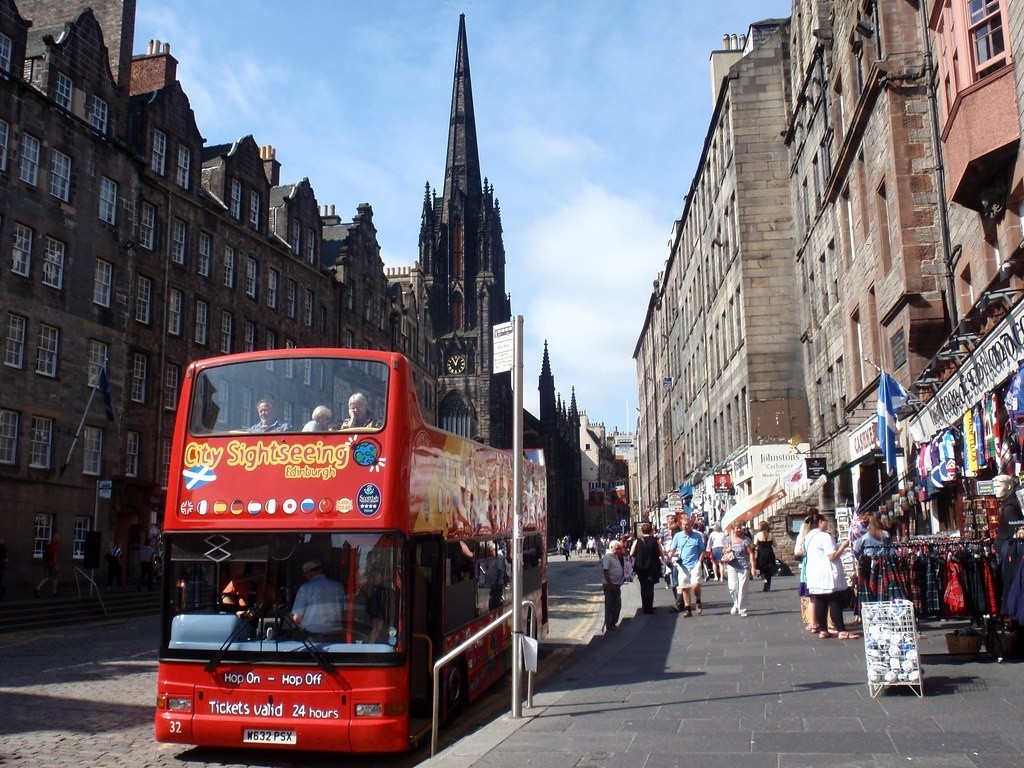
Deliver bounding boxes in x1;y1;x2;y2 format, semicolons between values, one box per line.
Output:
302;558;324;573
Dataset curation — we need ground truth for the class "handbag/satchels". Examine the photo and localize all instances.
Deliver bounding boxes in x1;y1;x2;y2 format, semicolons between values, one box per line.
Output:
720;551;737;564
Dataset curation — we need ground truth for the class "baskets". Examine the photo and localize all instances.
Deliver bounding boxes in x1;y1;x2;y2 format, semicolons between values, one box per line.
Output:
946;633;983;662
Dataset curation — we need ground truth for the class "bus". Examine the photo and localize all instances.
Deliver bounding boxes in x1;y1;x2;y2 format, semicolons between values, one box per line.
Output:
155;346;550;754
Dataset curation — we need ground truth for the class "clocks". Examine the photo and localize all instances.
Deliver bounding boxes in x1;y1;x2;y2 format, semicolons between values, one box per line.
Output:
448;356;466;374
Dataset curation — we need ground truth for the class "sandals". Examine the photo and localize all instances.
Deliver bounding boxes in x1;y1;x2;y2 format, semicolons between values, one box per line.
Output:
836;632;861;640
818;632;832;639
810;627;820;634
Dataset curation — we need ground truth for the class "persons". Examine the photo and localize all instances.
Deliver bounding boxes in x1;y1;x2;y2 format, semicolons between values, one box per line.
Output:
106;540;128;593
630;511;778;617
556;535;635;562
34;534;60;598
220;561;281;618
340;393;382;428
601;540;624;631
450;541;512;611
355;569;384;643
246;399;290;433
301;405;338;432
291;560;345;643
136;538;164;592
794;509;889;639
0;536;8;594
991;475;1024;555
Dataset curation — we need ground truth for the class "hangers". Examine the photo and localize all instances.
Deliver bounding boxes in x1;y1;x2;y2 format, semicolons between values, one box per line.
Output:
859;537;987;559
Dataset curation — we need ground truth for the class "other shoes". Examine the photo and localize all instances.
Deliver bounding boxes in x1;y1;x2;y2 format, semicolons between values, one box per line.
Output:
696;603;703;614
120;588;128;593
34;588;41;598
643;605;657;614
763;582;769;592
106;588;114;594
729;605;737;616
738;610;747;617
48;591;59;598
683;610;693;619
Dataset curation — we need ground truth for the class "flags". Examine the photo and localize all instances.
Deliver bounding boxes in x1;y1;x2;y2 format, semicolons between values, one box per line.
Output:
100;368;115;423
783;458;813;490
609;480;626;506
877;369;908;475
588;481;604;506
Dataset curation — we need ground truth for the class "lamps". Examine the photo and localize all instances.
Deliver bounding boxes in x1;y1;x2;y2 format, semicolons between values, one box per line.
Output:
830;399;879;435
914;259;1018;392
854;22;874;38
713;239;725;247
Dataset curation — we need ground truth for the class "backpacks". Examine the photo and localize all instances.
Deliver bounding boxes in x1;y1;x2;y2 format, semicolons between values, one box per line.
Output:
42;546;51;561
